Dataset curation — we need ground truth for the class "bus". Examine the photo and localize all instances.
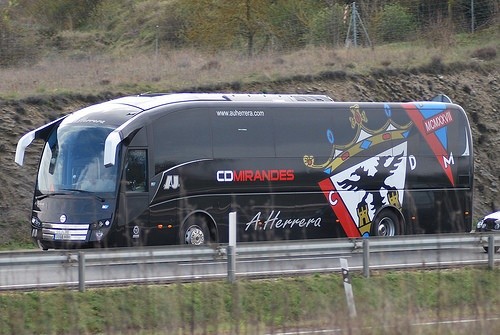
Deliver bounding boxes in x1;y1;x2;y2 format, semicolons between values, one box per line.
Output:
13;92;475;245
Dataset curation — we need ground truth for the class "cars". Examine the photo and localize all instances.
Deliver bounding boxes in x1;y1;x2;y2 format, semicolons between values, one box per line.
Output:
477;211;500;253
